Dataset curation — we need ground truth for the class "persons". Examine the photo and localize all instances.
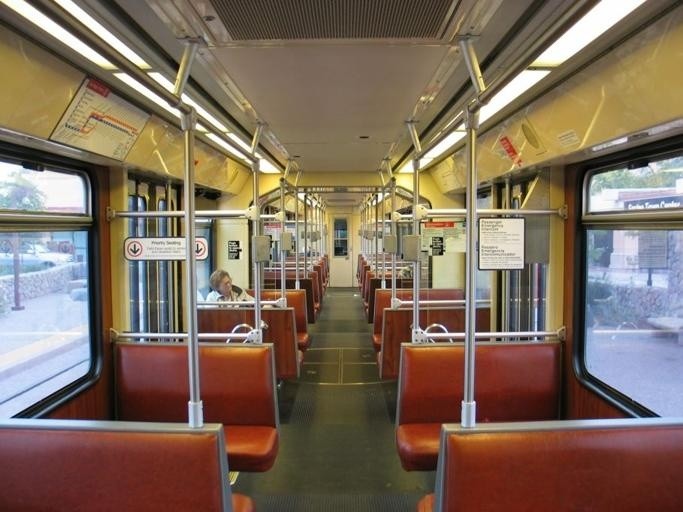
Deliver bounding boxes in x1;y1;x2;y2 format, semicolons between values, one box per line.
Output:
398;262;413;279
205;270;274;309
